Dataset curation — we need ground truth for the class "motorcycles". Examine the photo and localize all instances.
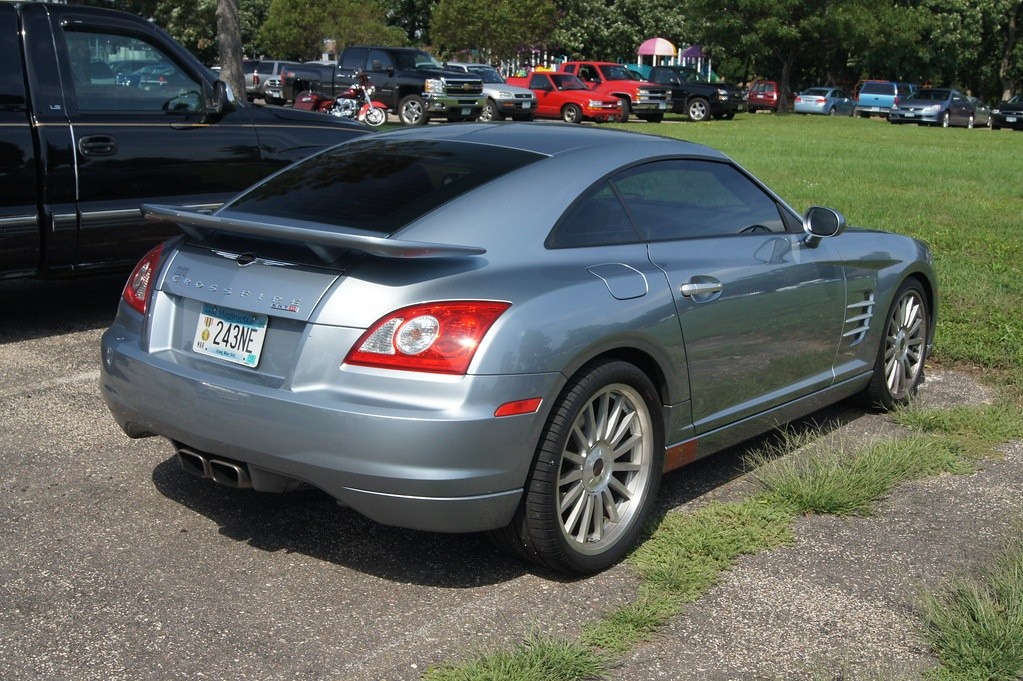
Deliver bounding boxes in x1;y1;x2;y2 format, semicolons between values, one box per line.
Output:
293;68;390;129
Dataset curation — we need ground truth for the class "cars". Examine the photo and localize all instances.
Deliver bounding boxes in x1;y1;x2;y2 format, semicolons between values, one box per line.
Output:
888;87;976;130
416;61;538;123
101;121;939;580
990;93;1023;130
794;87;855;118
960;94;994;127
210;58;300;108
747;80;799;114
847;79;889;110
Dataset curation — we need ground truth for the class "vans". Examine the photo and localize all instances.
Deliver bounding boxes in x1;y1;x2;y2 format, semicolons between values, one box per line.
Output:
855;81;923;122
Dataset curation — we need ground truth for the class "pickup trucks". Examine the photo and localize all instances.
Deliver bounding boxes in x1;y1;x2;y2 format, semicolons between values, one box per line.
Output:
506;69;624;126
279;44;488;129
0;0;379;320
642;65;740;122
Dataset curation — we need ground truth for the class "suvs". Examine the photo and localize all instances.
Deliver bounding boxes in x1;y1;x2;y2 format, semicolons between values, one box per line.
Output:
557;63;673;124
264;60;338;111
